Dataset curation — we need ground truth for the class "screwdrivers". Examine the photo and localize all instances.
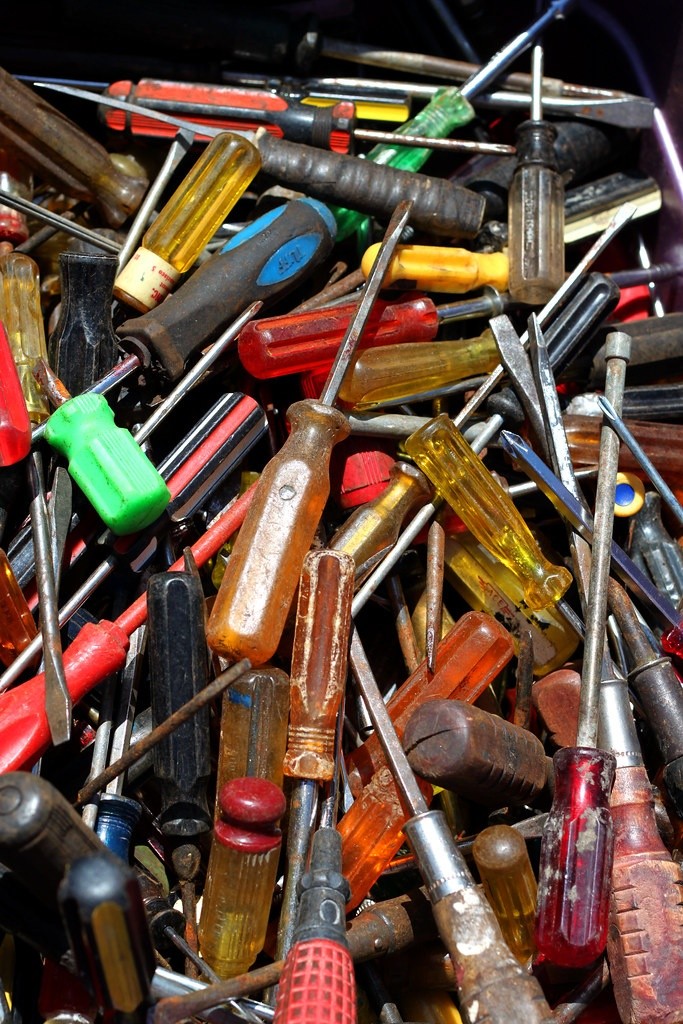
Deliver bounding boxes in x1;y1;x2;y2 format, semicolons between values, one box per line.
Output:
0;0;683;1024
202;199;419;657
